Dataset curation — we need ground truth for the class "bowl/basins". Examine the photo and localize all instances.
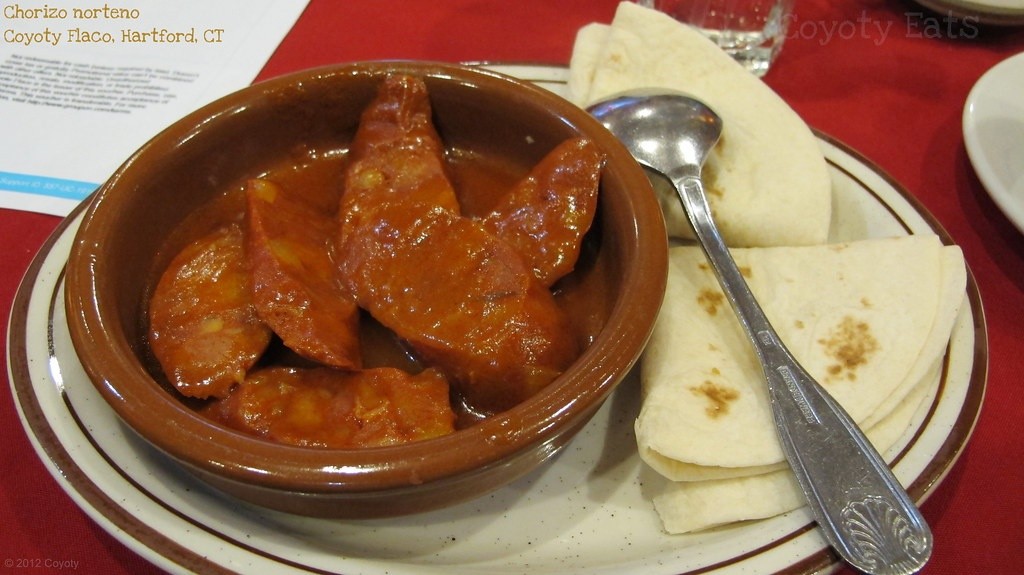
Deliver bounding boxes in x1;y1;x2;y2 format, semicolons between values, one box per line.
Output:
64;58;671;522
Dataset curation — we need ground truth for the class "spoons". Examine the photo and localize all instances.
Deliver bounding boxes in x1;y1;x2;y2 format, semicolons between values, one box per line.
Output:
584;84;933;575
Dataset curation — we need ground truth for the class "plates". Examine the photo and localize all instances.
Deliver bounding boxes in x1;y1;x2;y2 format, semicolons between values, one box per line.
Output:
5;65;991;575
961;52;1024;234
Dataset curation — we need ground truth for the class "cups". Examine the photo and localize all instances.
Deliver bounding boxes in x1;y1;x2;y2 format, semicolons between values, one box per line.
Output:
633;0;789;78
